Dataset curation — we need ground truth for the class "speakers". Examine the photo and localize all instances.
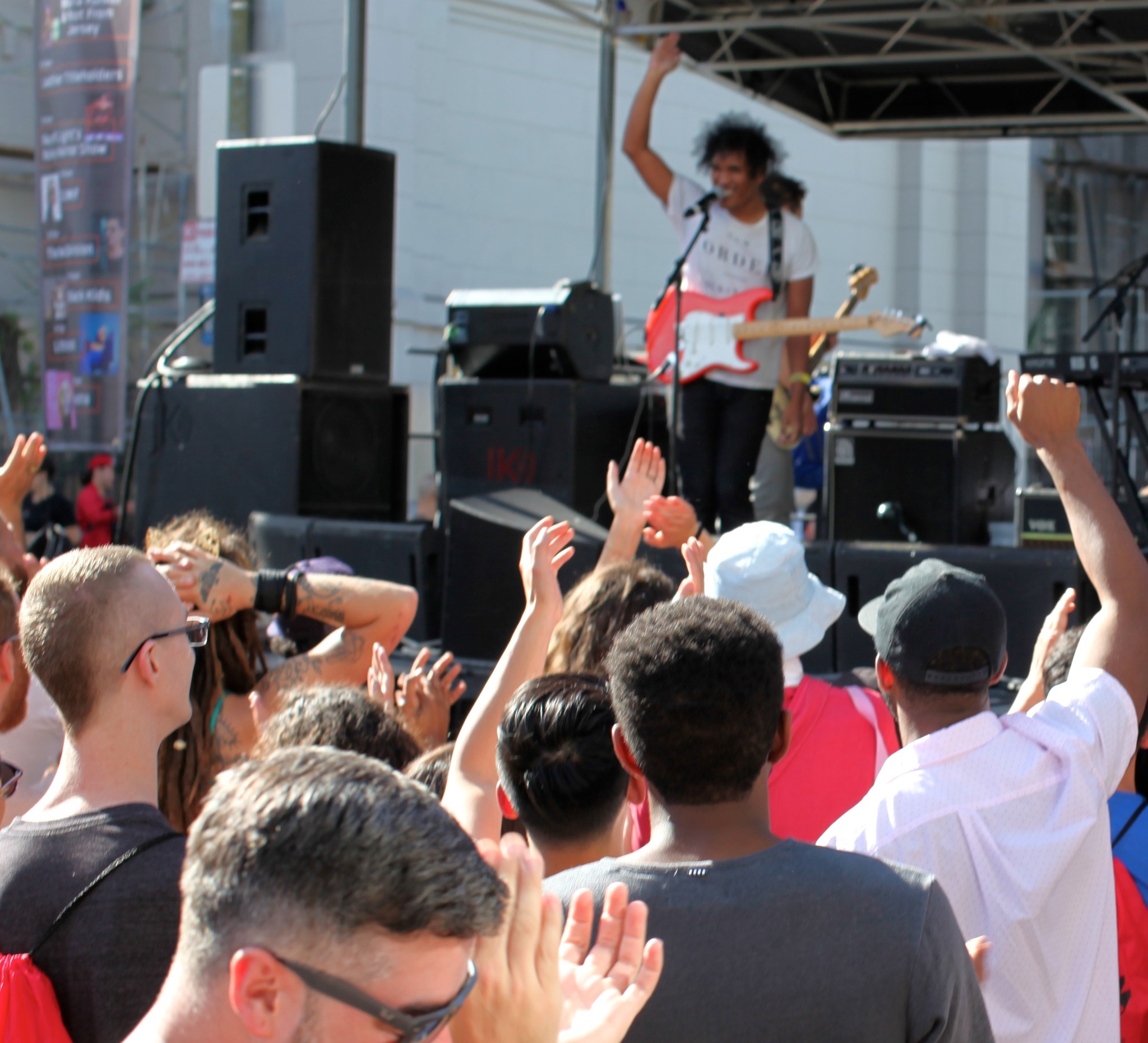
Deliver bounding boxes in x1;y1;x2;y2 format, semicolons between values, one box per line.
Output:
834;540;1099;672
133;135;835;674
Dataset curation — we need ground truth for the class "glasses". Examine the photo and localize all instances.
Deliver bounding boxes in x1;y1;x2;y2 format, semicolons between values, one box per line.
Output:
264;947;477;1043
123;615;207;675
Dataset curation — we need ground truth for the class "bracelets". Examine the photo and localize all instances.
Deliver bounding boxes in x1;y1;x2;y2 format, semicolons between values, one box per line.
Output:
286;568;303;623
253;568;288;613
694;522;704;542
790;371;812;385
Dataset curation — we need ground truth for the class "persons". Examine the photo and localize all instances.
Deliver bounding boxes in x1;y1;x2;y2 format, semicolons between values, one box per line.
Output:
0;369;1148;1043
621;37;841;533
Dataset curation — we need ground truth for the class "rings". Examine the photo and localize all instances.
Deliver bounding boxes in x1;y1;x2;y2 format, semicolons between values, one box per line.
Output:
648;473;655;482
29;465;38;474
20;465;30;472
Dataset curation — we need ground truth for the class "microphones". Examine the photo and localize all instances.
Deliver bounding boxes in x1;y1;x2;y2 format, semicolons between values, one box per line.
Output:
876;502;906;522
684;185;725;217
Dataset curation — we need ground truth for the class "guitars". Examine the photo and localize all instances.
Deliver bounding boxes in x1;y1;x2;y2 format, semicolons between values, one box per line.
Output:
646;287;934;386
766;262;878;449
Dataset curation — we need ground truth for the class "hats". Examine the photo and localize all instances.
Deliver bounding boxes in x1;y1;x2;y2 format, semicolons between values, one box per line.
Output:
88;455;113;470
701;520;847;659
858;555;1005;691
